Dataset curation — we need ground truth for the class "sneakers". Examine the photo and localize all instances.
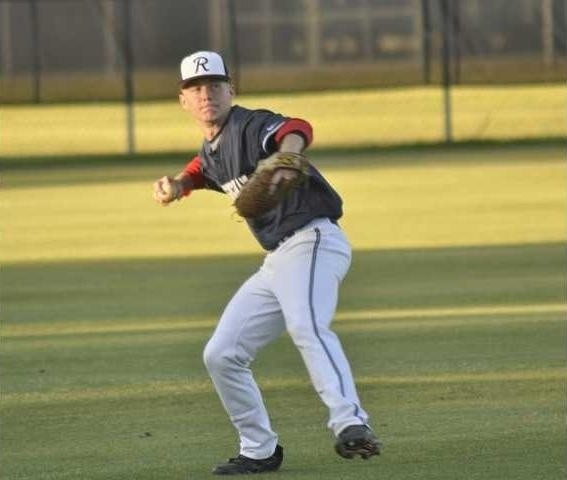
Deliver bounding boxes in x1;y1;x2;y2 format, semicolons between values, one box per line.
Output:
213;444;284;476
334;426;384;459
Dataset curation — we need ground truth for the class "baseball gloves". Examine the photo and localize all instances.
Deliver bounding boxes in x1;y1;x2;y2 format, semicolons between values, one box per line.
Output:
233;153;308;217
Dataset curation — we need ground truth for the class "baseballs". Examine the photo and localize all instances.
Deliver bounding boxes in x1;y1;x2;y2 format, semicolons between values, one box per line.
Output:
162;182;177;195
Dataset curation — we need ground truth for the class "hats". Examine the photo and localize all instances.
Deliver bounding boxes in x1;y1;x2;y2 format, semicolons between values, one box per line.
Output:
177;50;232;89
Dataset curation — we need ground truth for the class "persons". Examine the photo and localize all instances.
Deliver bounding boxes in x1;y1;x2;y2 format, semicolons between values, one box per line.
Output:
148;47;385;476
